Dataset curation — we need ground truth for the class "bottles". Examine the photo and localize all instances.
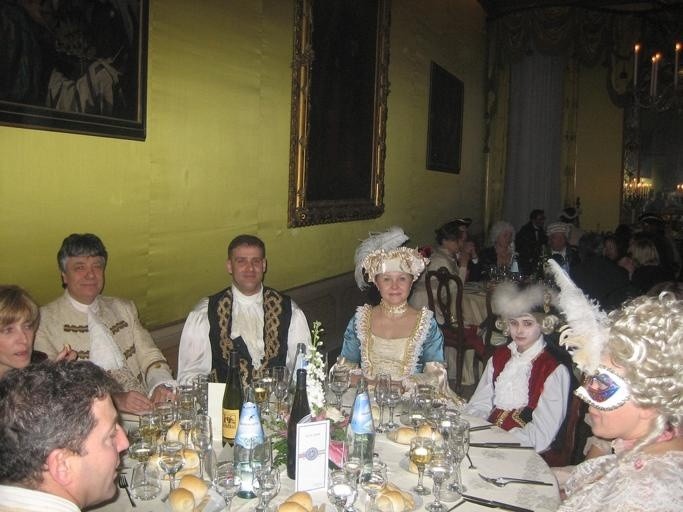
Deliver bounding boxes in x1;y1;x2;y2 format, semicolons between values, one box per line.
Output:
285;341;310;420
222;348;244;448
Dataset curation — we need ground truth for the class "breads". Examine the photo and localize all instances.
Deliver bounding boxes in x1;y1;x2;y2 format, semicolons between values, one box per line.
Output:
368;484;415;512
278;502;309;512
394;423;435;473
285;492;313;512
148;424;208;512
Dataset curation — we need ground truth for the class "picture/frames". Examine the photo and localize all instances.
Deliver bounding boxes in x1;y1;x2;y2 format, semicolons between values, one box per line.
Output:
0;0;150;141
285;1;392;229
423;59;462;174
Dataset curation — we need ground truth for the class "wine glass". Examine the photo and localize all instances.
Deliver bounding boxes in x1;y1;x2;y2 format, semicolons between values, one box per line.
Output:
485;263;559;292
125;363;469;512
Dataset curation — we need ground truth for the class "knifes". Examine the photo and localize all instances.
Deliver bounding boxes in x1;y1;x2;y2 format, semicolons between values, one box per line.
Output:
460;493;534;512
469;422;496;432
468;442;534;450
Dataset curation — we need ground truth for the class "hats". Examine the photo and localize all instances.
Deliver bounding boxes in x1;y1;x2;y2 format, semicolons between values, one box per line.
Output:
489;279;560;316
638;213;665;223
454;218;472;228
353;227;431;293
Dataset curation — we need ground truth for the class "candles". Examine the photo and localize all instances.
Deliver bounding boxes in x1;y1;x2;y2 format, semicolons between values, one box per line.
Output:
631;41;681;97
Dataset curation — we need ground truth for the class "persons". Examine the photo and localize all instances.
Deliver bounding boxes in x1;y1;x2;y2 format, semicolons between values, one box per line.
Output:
547;290;683;511
0;358;129;511
31;232;178;436
456;277;571;467
175;233;313;388
335;225;467;407
0;281;78;402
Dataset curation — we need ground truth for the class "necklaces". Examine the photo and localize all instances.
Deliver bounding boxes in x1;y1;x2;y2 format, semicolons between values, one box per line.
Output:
378;298;407;317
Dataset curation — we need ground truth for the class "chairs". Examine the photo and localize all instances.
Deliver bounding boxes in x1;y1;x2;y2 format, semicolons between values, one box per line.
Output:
536;390;585;466
423;266;482;397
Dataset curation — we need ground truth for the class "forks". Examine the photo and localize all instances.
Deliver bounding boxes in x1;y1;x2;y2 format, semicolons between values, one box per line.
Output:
477;473;554;488
116;475;137;508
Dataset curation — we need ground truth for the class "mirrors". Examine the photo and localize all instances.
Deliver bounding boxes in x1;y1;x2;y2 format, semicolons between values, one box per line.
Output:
617;55;681;236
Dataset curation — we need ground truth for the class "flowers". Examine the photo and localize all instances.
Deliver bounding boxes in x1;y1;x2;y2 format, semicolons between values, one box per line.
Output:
298;317;327;423
